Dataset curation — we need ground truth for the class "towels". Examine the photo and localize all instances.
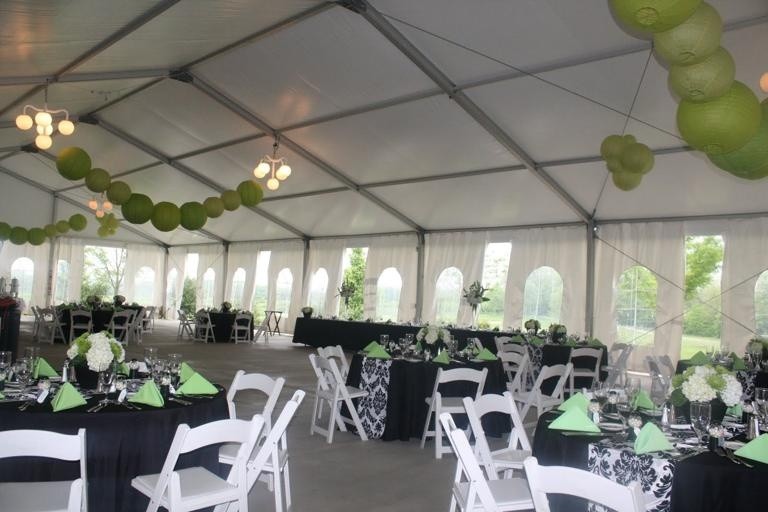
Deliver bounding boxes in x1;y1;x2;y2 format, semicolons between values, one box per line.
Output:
180;361;195;384
558;392;588;416
176;372;218;395
530;336;544;345
633;422;675;455
633;390;658;410
128;380;164;408
51;382;87;412
475;347;497;360
32;358;59;379
0;392;5;399
512;334;521;340
569;339;576;346
363;340;378;352
547;406;600;433
432;350;450;364
117;362;129;377
367;345;391;358
733;432;768;464
725;403;742;418
682;349;711;366
590;338;603;346
728;350;749;371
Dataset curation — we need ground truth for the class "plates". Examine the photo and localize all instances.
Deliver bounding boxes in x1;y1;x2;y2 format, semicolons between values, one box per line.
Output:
686;437;700;445
604;412;620;420
600;423;630;431
724;441;744;451
672;425;692;430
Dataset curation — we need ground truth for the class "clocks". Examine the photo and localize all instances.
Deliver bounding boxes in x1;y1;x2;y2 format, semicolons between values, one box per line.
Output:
669;366;742;407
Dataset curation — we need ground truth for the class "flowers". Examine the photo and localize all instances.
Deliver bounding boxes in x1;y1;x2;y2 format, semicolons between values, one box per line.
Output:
525;320;541;329
65;331;125;371
335;277;355;304
417;327;451;344
88;296;100;303
112;295;125;302
548;325;566;333
302;307;312;314
746;336;768;354
222;302;231;309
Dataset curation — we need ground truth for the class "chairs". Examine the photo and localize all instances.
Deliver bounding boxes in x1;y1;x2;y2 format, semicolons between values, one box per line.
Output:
645;356;675;406
128;414;265;512
145;305;157;331
31;306;49;338
657;353;678;384
448;391;532;479
603;345;633;387
138;309;150;343
216;389;307;512
496;341;538;391
561;348;604;402
176;311;195;339
194;312;215;344
307;356;369;442
229;315;253;344
222;372;287;491
421;366;488;460
507;362;573;443
318;346;349;419
524;458;643;512
253;313;273;345
37;305;67;345
122;310;138;344
465;337;483;353
0;427;88;512
70;308;94;347
105;308;130;347
440;414;532;512
496;351;536;414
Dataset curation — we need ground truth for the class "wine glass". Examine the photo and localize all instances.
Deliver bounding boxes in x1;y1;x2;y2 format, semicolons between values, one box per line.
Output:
624;377;641;405
691;400;712;456
167;353;182;370
15;358;32;401
380;335;388;345
24;347;40;360
593;380;610;407
654;401;675;434
152;358;167;387
145;345;159;369
406;334;414;345
399;338;406;360
0;351;12;370
99;364;116;405
449;334;455;341
449;340;457;357
467;337;475;357
616;397;635;438
756;387;768;419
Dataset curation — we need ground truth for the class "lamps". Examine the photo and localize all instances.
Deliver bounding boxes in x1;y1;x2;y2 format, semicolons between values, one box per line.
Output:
254;137;291;188
14;84;74;149
86;191;115;217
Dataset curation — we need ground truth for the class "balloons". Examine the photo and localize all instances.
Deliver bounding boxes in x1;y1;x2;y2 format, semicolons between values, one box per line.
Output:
95;212;119;238
599;131;657;194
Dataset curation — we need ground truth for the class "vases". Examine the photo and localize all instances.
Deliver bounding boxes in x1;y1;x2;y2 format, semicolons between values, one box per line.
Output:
681;398;728;424
464;282;488;311
115;302;122;307
75;367;113;388
552;334;561;342
345;297;350;320
472;306;479;331
91;304;98;308
527;329;536;335
304;313;310;318
751;354;767;363
223;309;228;313
422;340;444;353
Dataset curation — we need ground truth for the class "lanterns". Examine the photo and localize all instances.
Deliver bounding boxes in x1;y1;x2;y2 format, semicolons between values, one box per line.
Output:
760;98;767;115
107;181;131;206
664;48;737;102
707;114;768;181
8;225;28;244
238;179;262;208
222;191;241;211
28;228;46;246
150;201;181;231
71;214;88;232
606;0;703;33
121;192;152;223
0;222;12;242
205;196;225;218
57;220;70;234
652;2;724;67
86;168;112;194
55;145;91;180
179;202;207;231
44;225;57;239
673;78;762;155
